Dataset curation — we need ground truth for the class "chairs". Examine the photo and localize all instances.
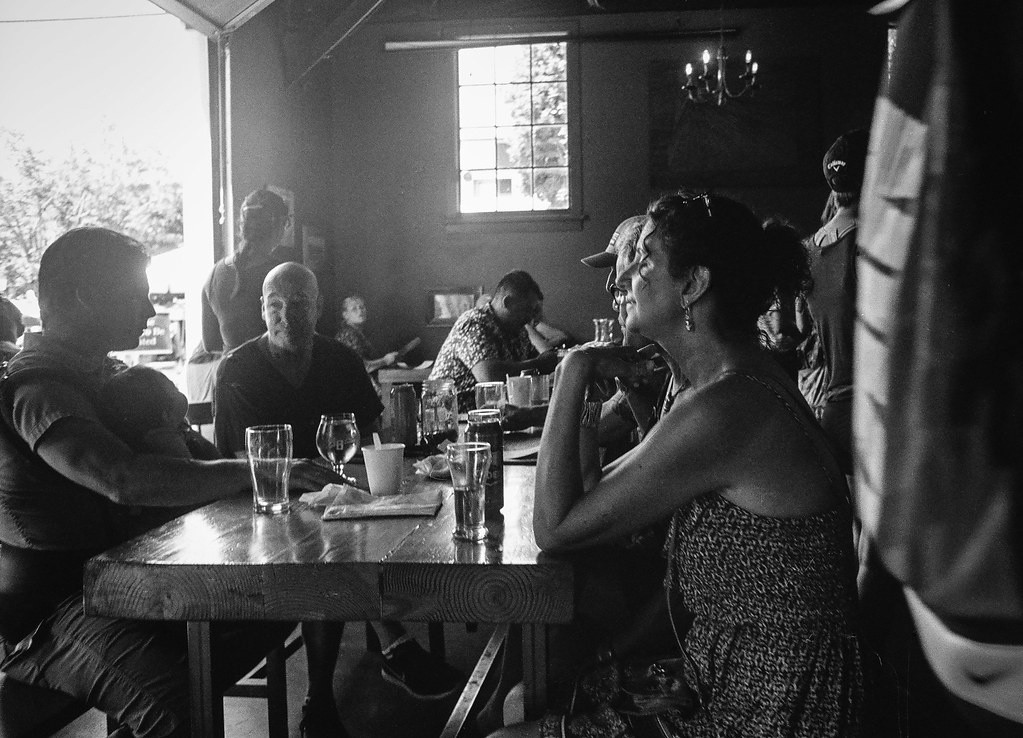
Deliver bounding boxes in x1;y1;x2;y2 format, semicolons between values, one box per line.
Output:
0;615;480;737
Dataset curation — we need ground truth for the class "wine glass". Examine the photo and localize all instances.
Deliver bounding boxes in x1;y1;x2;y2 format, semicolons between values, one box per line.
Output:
315;413;361;483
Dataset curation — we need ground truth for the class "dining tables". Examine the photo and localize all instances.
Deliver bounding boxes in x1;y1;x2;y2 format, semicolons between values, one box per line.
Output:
152;357;540;465
81;462;577;737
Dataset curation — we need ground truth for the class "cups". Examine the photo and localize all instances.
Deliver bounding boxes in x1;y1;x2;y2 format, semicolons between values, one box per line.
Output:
507;376;533;433
526;375;550;429
475;381;506;417
245;425;293;513
446;443;489;541
468;409;501;419
362;443;406;497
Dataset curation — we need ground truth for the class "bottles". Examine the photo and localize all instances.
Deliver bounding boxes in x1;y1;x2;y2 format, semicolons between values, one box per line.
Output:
519;368;540;378
592;317;616;342
421;378;460;447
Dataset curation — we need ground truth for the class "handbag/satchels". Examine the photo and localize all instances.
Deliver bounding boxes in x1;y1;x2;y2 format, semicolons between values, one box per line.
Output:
618;655;698;719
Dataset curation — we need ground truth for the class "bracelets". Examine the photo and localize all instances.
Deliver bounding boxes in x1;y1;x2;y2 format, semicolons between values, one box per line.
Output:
577;397;602;429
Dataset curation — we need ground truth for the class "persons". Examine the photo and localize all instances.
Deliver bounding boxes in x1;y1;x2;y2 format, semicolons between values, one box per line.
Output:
0;225;355;737
573;216;674;664
201;187;303;359
336;294;408;394
794;128;886;602
423;268;575;423
213;262;471;737
487;186;908;738
106;361;206;528
0;296;26;377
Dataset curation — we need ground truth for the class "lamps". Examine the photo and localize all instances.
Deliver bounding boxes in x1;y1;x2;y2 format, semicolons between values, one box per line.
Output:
680;0;759;109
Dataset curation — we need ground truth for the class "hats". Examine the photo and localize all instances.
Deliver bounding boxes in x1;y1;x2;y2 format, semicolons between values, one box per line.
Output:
580;215;649;269
822;122;869;193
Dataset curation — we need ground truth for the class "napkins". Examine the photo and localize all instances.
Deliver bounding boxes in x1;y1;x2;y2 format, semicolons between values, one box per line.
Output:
412;453;451;481
321;483;443;521
298;476;343;508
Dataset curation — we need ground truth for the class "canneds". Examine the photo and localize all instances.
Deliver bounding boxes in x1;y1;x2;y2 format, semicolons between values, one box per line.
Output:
463;408;505;514
388;384;417;445
